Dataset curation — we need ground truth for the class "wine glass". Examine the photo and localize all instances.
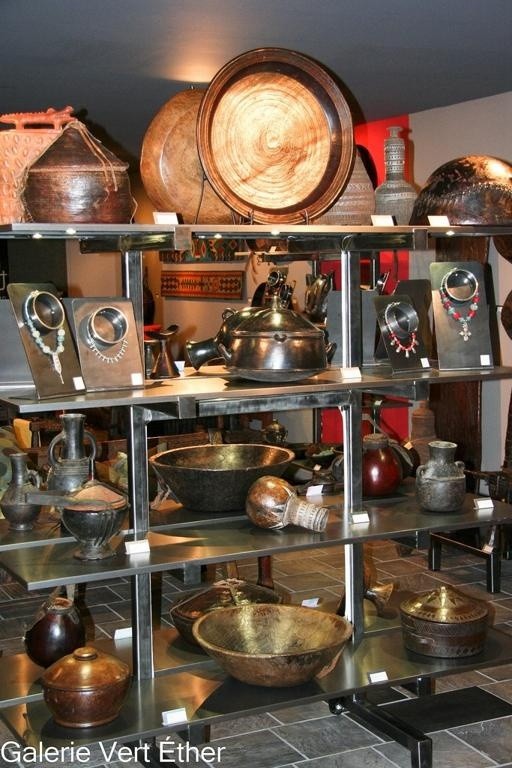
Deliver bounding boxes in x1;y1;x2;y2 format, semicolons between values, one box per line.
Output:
61;503;129;561
335;560;394;618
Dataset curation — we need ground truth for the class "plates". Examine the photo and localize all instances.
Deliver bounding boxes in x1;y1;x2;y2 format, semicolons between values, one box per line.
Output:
140;47;354;225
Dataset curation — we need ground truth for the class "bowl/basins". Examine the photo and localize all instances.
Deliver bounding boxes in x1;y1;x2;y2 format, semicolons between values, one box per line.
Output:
193;603;353;688
150;444;296;512
37;675;131;726
170;596;283;647
23;169;132;224
306;450;335;467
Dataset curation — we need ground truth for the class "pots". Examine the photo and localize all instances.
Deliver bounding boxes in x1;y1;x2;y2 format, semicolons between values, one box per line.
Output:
399;585;489;659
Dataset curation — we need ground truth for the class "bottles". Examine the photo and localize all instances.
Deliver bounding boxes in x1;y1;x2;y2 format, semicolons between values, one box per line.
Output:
414;442;468;513
361;432;405;499
25;598;84;665
186;307;269;372
372;125;421;227
0;412;98;533
245;475;330;533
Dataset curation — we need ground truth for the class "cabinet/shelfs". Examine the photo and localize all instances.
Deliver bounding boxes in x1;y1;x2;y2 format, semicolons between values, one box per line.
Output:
3;212;512;768
